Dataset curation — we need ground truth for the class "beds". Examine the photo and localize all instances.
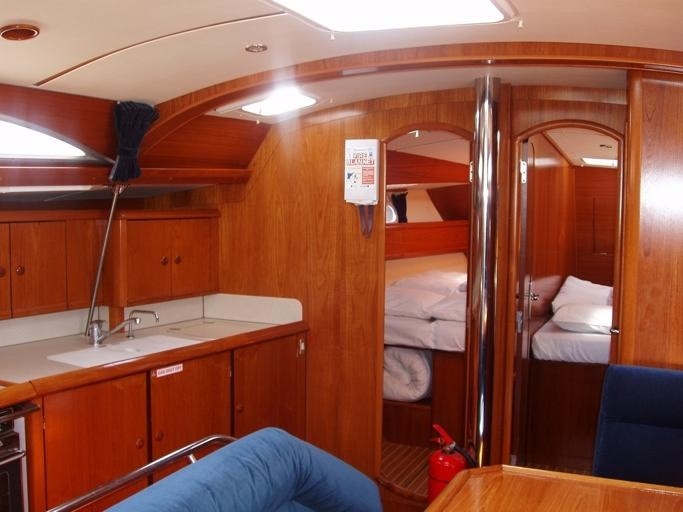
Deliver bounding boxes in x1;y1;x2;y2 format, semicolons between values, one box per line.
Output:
380;249;462;456
527;318;612;472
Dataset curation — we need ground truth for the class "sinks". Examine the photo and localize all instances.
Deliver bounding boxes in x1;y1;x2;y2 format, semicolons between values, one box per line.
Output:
46;347;136;368
118;336;204;352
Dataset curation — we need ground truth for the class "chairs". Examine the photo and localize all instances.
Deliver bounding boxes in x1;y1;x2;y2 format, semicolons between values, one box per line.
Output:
590;362;683;486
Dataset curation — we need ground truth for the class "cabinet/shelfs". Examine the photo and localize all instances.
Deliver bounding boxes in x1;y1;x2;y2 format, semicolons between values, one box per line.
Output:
234;332;307;444
37;349;234;507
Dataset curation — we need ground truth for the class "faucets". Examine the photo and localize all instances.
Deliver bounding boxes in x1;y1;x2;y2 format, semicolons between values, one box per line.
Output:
128;310;160;336
90;317;141;348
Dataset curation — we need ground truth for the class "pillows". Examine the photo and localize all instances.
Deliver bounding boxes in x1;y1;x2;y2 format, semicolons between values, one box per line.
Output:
383;269;466;323
548;273;616;335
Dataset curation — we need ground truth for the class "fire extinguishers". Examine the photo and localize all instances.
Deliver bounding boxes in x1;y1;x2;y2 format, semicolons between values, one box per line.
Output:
427;423;478;507
